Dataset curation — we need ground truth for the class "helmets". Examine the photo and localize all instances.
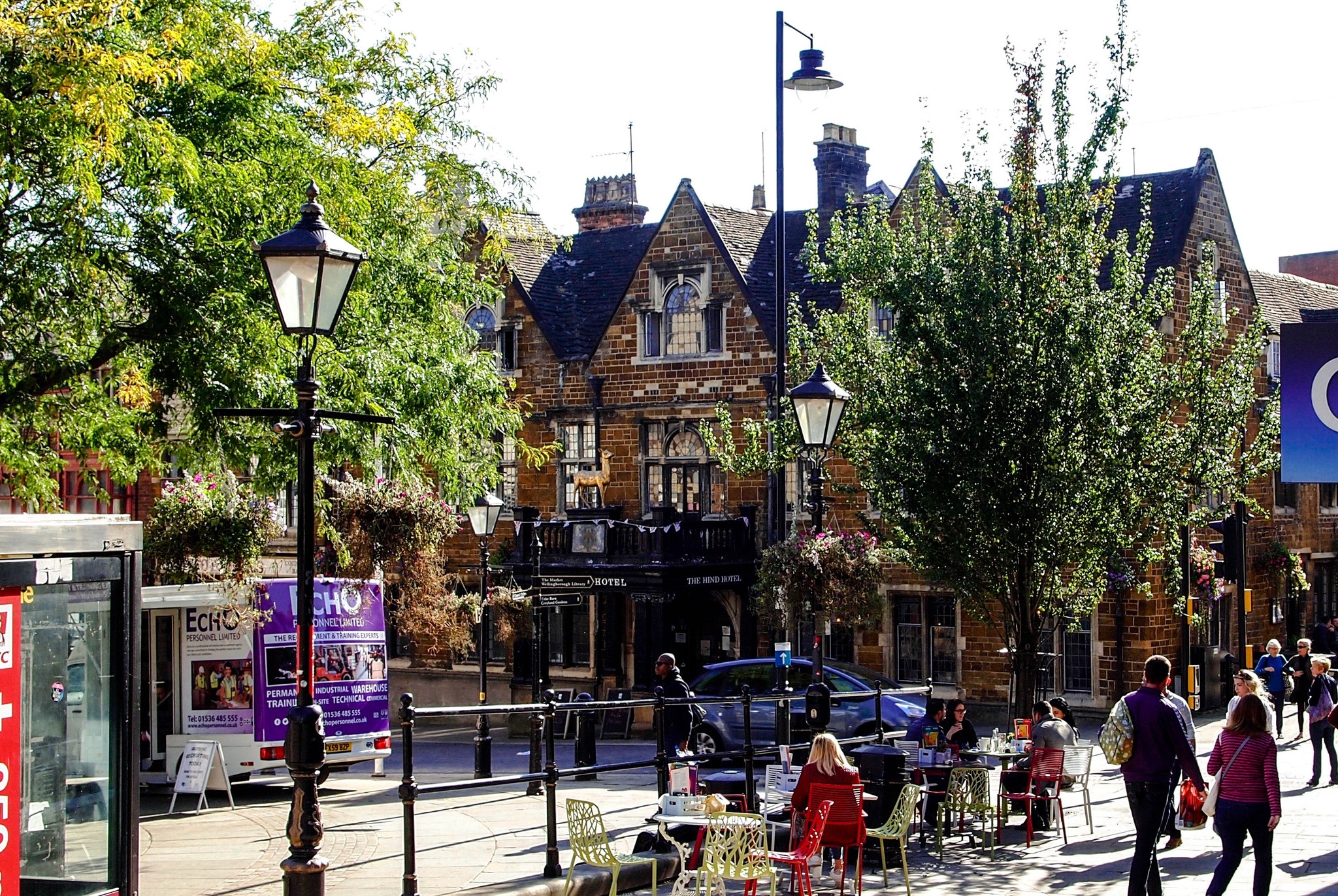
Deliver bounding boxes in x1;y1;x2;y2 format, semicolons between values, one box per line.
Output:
199;666;204;673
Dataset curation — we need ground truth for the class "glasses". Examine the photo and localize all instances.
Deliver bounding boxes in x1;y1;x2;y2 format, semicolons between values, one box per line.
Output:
655;661;670;667
1236;671;1252;682
1269;647;1279;651
955;709;967;715
1296;645;1307;649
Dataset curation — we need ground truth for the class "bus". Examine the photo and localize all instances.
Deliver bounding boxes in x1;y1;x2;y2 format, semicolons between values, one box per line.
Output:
142;573;393;794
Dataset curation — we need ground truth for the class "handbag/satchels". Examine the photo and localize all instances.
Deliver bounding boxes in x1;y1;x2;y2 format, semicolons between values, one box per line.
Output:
1176;780;1210;830
1310;676;1333;723
1202;766;1222;816
1098;696;1135;764
1283;674;1295;695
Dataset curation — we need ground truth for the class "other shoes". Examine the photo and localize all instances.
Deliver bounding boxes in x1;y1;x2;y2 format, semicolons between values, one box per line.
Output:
1278;735;1283;738
915;802;938;826
645;811;659;822
1306;778;1319;786
1295;736;1302;740
1329;778;1338;785
1014;816;1042;830
1165;837;1182;848
974;814;989;822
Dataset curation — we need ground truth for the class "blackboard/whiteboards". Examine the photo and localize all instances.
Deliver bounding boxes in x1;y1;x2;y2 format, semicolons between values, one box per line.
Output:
174;740;232;793
601;688;633;736
545;688;577;736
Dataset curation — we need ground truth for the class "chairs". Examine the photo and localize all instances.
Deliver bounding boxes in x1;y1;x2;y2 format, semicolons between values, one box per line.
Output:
562;739;1096;896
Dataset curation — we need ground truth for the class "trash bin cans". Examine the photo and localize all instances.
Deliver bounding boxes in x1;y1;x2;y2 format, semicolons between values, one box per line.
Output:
849;745;910;847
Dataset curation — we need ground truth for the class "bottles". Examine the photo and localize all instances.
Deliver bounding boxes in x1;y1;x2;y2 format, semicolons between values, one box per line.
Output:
675;746;697;765
991;729;998;753
995;728;1000;747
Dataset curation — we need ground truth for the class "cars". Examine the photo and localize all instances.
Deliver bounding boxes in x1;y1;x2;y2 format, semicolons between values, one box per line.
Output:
685;654;946;770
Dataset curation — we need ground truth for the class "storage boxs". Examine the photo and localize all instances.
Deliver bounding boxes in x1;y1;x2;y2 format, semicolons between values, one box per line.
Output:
660;793;708;817
774;771;801;792
1009;738;1033;753
918;746;952;766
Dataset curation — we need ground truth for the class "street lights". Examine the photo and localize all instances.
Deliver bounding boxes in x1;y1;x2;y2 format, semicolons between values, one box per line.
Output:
775;6;846;763
211;179;399;896
787;362;854;763
465;483;507;779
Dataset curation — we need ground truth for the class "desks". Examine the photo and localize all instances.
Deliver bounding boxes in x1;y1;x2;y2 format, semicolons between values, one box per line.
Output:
654;809;761;896
766;785;880;896
957;744;1031;830
905;757;997;850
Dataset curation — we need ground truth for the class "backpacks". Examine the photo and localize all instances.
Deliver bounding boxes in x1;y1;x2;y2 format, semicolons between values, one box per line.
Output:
669;679;707;728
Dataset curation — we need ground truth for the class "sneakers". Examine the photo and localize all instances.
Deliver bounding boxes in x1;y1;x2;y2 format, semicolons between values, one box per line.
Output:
830;866;843;882
807;856;822;866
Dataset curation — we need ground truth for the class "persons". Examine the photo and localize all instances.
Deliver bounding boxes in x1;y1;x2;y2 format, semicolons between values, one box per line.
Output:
1225;669;1275;742
645;652;693;823
1253;638;1290;741
1201;693;1281;896
1282;638;1314;741
1047;697;1080;822
791;733;866;882
939;698;990;822
1098;655;1210;896
192;659;252;710
316;643;389;681
902;698;962;828
1309;614;1338;681
139;683;174;771
999;700;1078;834
1306;656;1338;787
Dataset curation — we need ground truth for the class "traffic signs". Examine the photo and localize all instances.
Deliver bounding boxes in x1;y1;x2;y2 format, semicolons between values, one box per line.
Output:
530;575;594;589
532;592;583;608
510;588;536;601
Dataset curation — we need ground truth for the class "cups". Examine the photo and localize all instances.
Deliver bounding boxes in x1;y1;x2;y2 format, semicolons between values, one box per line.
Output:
976;739;987;751
1010;746;1016;752
981;737;990;750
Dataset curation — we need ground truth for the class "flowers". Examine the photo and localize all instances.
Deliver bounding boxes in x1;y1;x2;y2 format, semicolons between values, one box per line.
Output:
1104;563;1131;598
746;499;913;640
143;467;280;636
1184;535;1226;647
1261;538;1313;624
331;472;533;663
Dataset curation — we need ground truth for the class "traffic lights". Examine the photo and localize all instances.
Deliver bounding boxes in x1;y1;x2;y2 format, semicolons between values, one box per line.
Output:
1208;513;1236;582
